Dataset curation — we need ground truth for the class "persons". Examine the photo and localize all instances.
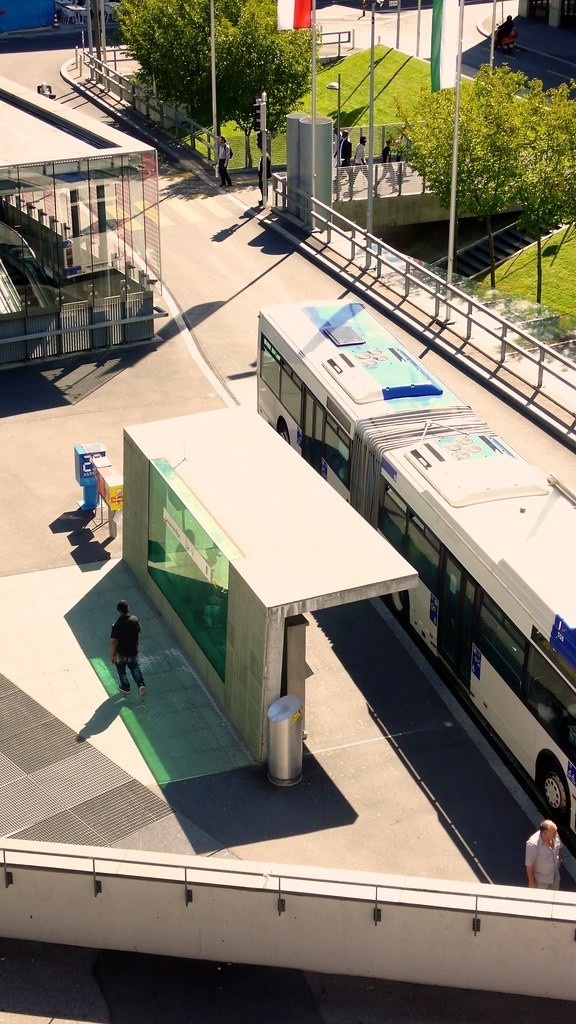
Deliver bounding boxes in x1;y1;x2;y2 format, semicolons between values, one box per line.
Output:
395;126;414;173
174;529;195;566
494;15;514;50
373;140;399;192
349;136;374;188
258;147;273;206
333;127;354;187
525;819;563;891
109;600;148;697
218;136;233;187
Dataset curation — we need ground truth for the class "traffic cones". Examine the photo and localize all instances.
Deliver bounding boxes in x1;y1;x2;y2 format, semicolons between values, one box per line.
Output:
52;14;61;30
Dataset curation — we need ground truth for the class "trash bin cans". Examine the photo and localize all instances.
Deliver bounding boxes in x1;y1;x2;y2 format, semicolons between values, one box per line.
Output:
266;693;305;790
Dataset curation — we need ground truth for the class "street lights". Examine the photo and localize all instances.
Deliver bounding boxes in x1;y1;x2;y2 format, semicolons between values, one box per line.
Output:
326;82;340;200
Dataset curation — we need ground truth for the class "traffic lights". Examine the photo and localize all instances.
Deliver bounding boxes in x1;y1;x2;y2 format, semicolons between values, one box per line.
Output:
252;103;260;132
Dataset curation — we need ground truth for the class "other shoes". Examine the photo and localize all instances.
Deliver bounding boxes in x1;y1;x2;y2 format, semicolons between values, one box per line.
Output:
226;183;233;187
219;183;226;187
392;189;399;192
118;685;130;694
373;186;376;191
137;682;148;698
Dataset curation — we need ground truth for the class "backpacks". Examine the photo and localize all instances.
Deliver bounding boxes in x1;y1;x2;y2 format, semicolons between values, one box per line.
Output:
224;145;233;159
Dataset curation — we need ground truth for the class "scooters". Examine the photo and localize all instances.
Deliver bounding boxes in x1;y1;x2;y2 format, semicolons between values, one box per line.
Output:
491;23;518;50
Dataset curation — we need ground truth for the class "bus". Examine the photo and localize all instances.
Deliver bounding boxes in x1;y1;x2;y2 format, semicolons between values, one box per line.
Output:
254;301;576;841
1;169;121;284
